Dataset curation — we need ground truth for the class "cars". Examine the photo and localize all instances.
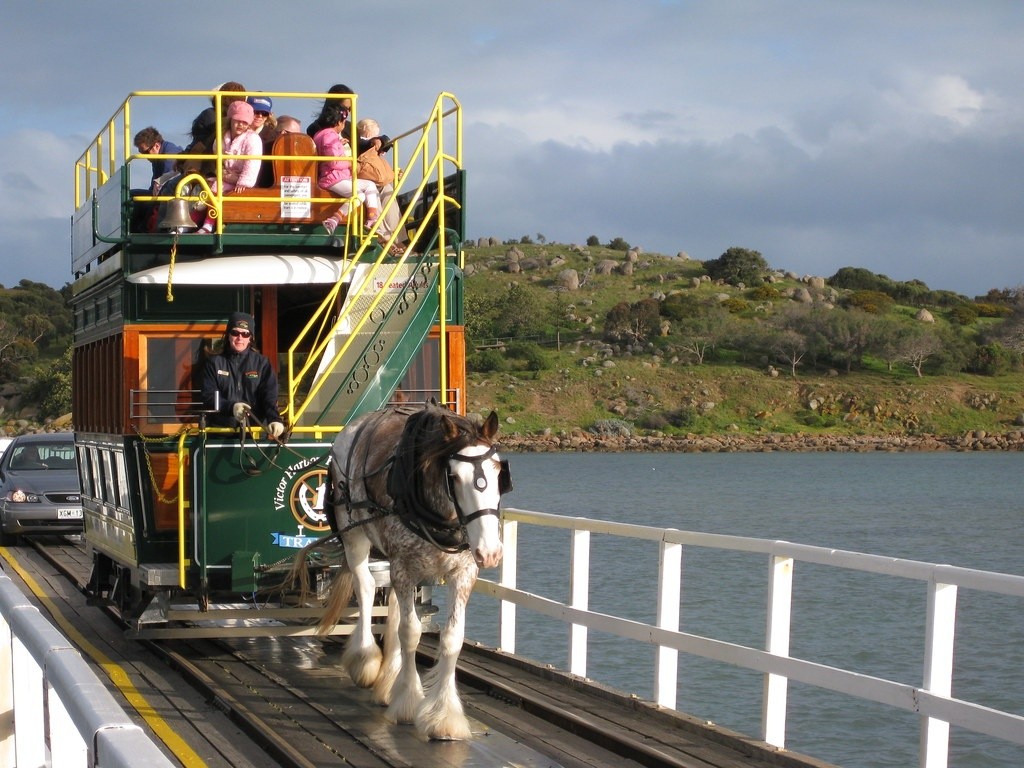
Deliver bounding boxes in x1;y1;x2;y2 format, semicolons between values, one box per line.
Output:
0;433;83;534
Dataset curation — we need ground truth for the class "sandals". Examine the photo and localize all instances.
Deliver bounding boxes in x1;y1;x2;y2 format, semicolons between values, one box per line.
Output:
364;219;386;239
322;217;338;236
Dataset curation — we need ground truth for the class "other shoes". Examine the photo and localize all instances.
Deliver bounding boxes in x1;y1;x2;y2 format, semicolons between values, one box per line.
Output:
194;228;215;235
376;235;408;258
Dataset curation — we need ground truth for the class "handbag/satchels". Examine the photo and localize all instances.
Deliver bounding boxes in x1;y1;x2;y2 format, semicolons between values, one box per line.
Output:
358;146;395;186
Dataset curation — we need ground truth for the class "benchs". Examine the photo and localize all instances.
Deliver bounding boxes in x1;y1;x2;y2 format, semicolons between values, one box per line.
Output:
223;132;366;224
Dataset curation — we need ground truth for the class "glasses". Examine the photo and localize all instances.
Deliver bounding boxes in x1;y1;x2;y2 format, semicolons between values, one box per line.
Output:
138;145;153;154
255;110;270;118
229;329;252;338
338;105;351;112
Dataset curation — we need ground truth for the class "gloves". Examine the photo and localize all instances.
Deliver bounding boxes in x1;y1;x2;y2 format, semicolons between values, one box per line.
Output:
268;421;284;437
232;403;252;423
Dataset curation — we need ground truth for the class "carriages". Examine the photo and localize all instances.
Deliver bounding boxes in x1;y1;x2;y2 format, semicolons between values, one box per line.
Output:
67;87;506;742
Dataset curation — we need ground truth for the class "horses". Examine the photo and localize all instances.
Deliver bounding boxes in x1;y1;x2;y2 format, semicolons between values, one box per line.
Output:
281;395;506;742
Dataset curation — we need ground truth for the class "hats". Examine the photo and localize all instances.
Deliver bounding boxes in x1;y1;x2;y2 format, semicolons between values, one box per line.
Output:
247;91;273;114
228;312;255;332
226;101;256;125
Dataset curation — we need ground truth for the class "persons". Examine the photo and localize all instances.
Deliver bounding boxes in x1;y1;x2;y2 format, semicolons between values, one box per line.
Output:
198;313;284;441
146;363;176;423
21;444;49;469
133;82;417;258
